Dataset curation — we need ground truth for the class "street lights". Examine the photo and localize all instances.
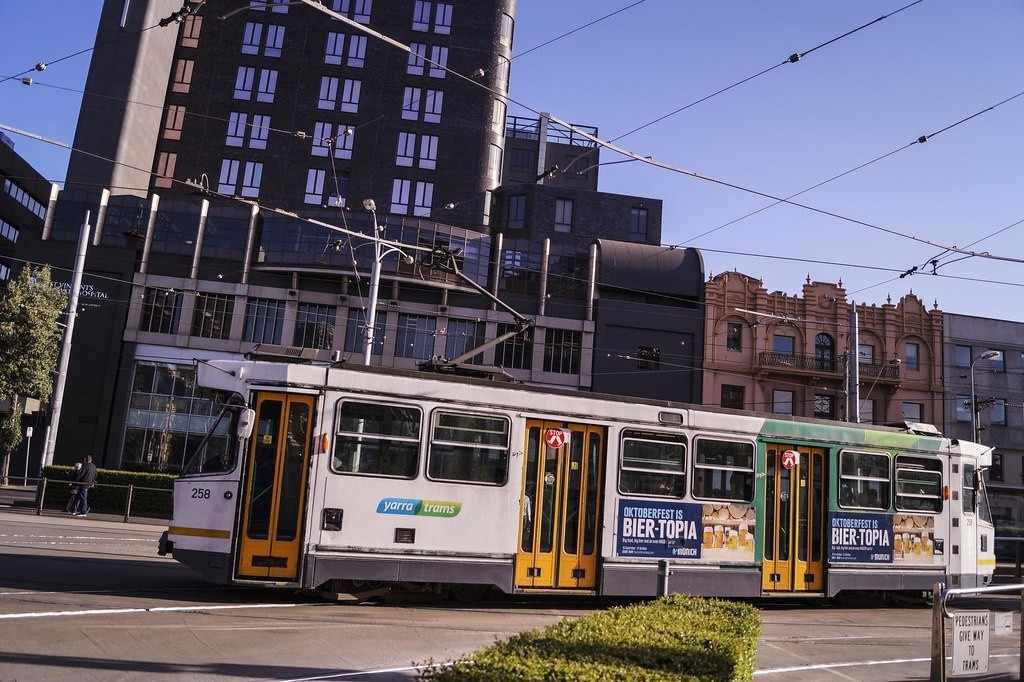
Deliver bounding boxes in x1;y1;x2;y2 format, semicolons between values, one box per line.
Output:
354;198;414;367
969;351;1001;444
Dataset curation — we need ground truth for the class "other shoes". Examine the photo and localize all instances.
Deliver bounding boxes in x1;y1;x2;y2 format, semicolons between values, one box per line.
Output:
76;513;86;517
72;512;76;515
61;511;70;514
86;506;90;513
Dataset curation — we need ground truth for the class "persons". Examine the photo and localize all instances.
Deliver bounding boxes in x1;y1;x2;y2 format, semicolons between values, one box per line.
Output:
69;455;97;517
841;484;856;506
523;495;531;536
61;463;90;514
867;490;879;507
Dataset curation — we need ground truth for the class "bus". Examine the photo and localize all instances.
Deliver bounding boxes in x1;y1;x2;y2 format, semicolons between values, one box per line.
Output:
167;359;996;601
